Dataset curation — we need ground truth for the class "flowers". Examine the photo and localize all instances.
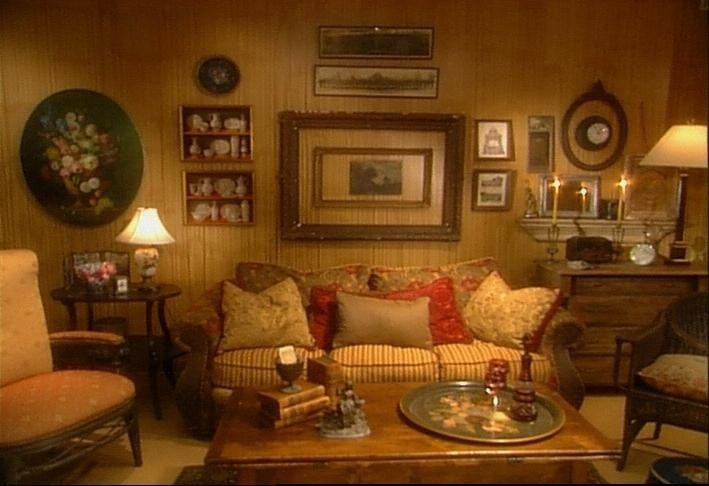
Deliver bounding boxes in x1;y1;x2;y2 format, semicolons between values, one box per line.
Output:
38;112;124;214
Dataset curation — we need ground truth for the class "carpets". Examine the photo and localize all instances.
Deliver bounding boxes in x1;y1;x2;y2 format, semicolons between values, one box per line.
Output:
174;459;612;486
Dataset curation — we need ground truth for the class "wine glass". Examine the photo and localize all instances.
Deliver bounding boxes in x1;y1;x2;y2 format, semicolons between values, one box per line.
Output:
275;355;305;395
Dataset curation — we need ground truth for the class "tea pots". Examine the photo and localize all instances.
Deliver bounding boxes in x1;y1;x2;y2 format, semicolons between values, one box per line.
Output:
233;175;250;198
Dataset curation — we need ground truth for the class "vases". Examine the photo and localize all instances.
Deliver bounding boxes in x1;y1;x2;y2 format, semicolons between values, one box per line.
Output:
60;177;81;212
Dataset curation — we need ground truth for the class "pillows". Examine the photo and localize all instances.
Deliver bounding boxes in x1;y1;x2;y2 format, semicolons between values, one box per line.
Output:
464;269;563;351
372;256;495;312
217;277;316;352
310;278;473;346
235;262;372;311
331;290;434;349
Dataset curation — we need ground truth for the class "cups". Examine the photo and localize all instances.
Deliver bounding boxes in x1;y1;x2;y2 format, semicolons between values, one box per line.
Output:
509;386;539;420
482;357;512;394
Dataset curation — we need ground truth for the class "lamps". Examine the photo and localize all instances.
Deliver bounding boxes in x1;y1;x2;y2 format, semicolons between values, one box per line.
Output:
637;119;709;266
110;206;178;296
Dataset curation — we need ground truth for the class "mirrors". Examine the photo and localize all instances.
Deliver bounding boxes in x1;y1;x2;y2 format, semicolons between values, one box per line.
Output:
536;175;601;220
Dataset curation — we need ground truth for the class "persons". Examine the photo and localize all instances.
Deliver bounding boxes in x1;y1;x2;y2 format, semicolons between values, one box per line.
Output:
482;128;504;155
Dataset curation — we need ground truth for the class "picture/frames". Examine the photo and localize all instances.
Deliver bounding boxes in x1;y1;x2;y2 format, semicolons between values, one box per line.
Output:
471;167;512;213
317;24;435;60
313;64;442;100
178;103;255;164
180;168;258;229
472;119;514;162
622;153;683;222
278;109;468;244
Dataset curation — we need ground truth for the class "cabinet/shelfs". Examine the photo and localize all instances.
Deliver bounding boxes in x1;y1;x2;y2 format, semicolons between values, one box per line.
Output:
529;259;709;391
48;283;192;418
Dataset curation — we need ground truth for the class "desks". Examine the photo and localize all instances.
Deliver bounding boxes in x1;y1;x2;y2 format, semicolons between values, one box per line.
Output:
204;382;624;485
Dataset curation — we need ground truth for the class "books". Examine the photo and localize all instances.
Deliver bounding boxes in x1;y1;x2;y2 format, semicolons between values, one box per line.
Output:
255;381;331;429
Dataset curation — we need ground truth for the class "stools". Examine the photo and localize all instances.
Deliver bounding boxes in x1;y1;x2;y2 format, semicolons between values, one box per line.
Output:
644;457;709;485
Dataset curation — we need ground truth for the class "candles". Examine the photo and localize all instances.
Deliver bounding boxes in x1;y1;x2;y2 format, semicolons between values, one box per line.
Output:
578;188;587;212
614;179;626;226
550;181;560;223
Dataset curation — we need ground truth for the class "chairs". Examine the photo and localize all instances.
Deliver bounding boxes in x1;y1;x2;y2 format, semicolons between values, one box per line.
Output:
0;247;145;486
610;293;709;472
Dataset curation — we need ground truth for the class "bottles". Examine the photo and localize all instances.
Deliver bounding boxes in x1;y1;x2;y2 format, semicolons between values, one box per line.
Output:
238;113;247;133
240;199;251;222
208;112;223;131
188;137;202;156
199;177;212;198
210;201;220;222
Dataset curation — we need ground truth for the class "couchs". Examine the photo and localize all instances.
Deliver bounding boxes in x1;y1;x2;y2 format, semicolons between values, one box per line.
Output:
168;260;588;442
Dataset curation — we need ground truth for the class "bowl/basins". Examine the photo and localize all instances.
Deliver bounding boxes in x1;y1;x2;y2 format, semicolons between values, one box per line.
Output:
203;149;214;158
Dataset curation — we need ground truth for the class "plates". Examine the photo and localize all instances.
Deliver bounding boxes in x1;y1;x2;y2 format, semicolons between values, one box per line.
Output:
214;178;236;198
186;114;201;130
220;203;240;221
629;242;656;265
210;139;230;156
224;118;241;129
